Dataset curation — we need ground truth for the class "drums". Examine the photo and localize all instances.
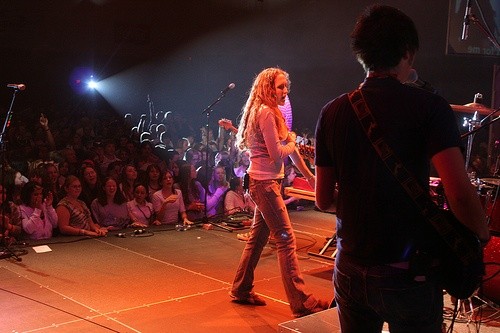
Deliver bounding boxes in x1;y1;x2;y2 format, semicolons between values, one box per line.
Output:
428;176;448;210
474;176;500;237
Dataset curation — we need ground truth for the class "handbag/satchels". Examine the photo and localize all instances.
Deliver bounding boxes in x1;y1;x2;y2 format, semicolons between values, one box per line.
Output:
347;88;486;300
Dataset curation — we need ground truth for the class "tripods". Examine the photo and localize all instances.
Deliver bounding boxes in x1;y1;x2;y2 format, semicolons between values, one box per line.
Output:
0;90;22;261
182;94;233;232
442;107;500;333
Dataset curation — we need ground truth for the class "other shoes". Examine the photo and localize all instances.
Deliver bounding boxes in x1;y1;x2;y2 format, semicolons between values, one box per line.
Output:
293;300;329;318
229;292;266;306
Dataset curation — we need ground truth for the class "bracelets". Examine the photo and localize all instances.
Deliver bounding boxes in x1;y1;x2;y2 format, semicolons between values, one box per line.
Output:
305;174;313;182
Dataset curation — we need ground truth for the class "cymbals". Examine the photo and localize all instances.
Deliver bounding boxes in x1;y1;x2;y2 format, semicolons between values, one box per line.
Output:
449;103;500;116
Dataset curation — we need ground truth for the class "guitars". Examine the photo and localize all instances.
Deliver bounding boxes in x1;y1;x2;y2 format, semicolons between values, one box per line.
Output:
438;208;485;299
218;119;244;137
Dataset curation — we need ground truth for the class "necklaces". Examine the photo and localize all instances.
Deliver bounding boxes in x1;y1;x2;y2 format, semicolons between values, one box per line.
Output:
136;205;152;219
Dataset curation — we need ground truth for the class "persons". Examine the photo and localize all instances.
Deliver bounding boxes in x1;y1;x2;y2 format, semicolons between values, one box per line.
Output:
468;139;488;178
0;112;319;246
229;68;328;319
314;4;490;333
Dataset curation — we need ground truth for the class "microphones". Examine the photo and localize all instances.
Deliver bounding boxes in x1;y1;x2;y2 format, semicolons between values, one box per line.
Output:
221;83;235;94
7;84;25;90
469;93;482;137
406;69;435;93
461;0;473;40
